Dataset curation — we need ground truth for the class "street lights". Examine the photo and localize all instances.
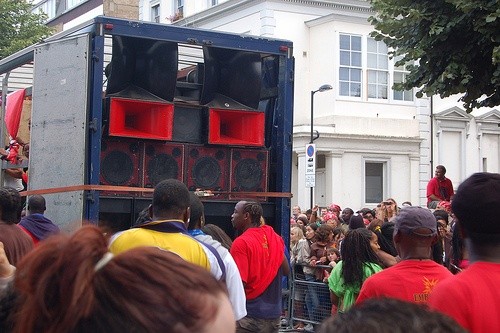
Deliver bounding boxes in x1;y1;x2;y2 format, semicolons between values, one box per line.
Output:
310;84;333;209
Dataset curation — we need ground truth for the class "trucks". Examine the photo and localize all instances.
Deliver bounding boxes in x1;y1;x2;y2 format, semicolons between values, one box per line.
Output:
0;15;295;300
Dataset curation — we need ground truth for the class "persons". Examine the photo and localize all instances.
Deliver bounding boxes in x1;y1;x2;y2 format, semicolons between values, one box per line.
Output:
426;165;454;208
230;200;290;333
290;198;470;333
0;143;248;333
427;173;500;333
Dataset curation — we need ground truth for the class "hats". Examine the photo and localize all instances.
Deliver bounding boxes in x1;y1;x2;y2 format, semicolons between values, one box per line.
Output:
330;204;342;211
451;172;500;231
395;206;438;238
323;211;338;221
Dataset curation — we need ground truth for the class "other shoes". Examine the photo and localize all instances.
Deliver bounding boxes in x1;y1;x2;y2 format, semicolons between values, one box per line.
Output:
281;317;321;332
